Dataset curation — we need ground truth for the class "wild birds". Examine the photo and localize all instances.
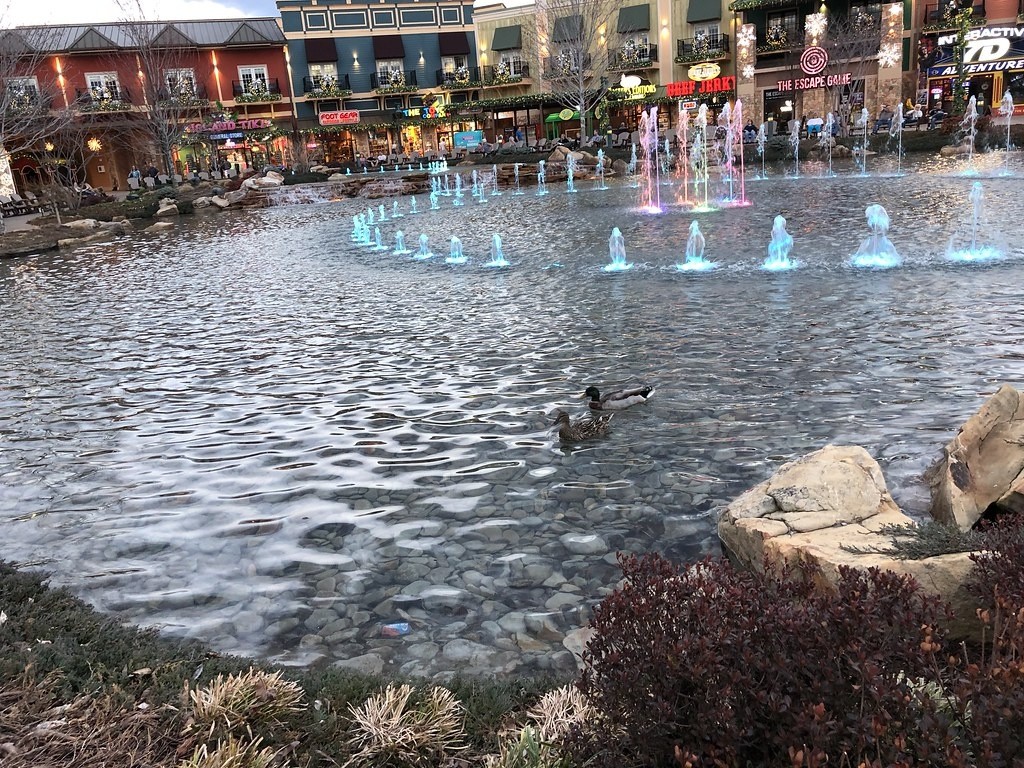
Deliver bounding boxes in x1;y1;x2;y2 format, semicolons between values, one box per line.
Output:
577;378;656;412
551;409;616;443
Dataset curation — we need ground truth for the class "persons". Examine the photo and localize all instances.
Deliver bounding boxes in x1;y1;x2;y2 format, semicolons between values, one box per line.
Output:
806;113;826;140
502;137;516;149
871;104;895;135
902;104;923;133
552;134;569;155
495;132;509;148
441;138;446;151
619;122;626;129
73;181;103;204
516;127;522;147
742;119;758;143
270;152;278;165
586;129;600;148
128;165;141;189
833;111;849;139
928;101;948;132
358;150;386;167
476;139;492;157
142;162;160;188
574;133;581;150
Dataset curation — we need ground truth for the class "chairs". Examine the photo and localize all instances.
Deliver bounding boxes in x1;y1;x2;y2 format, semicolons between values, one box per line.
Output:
806;124;824;139
764;121;778;139
701;126;716;147
547;137;571;151
742;129;759;144
381;138;547;167
876;118;892;133
589;135;603;148
683;127;697;148
625;131;641;151
927;115;946;130
713;126;727;141
659;128;678;149
571;136;589;149
600;133;617;150
786;119;802;140
0;162;302;218
612;132;630;151
849;113;869;136
905;117;922;131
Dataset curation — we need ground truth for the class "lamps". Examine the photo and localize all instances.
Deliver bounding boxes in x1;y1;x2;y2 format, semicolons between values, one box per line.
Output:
481;50;486;55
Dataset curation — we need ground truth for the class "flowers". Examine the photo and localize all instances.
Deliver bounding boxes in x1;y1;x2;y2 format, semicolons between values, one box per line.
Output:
1017;12;1024;21
922;16;987;31
